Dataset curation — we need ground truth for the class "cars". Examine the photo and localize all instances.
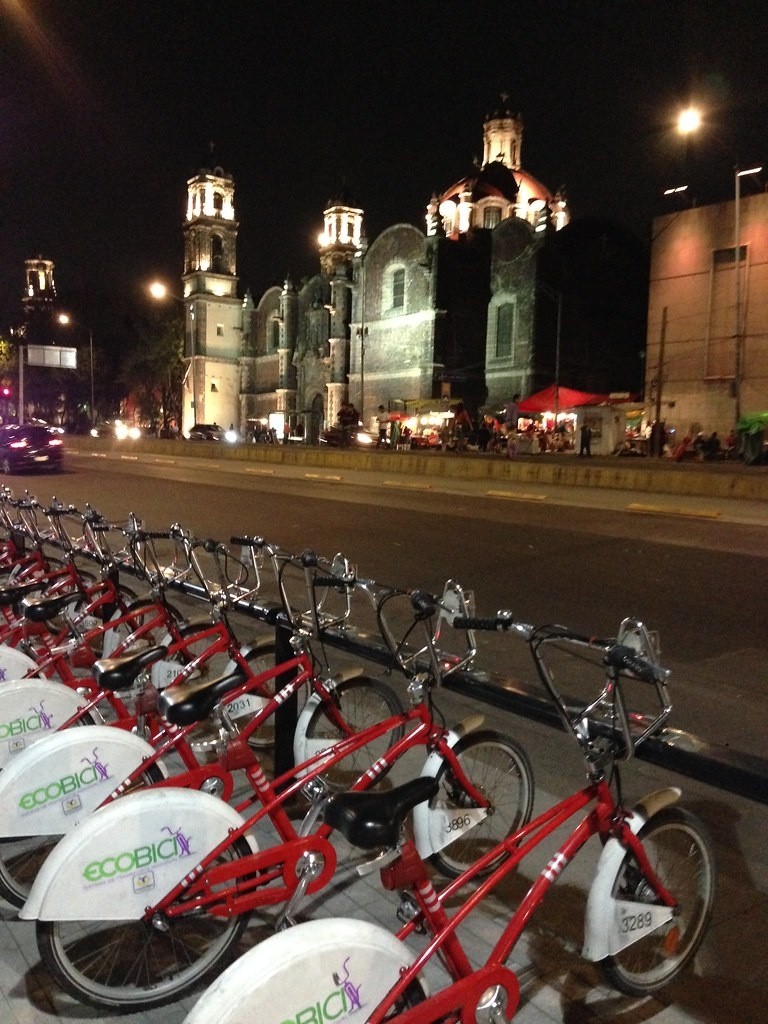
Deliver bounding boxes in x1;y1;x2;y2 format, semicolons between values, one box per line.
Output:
91;419;138;441
0;424;63;474
189;424;235;445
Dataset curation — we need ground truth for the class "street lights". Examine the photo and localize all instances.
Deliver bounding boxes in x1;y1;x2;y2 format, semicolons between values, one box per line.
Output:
59;314;94;426
319;233;368;427
675;107;742;425
151;282;197;428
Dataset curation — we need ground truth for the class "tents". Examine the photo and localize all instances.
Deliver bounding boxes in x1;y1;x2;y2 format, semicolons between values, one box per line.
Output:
514;384;636;414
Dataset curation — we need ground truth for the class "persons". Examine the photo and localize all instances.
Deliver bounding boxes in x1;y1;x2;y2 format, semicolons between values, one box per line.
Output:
296;421;304;443
468;421;495;454
507;394;521;458
254;426;261;443
579;420;593;457
403;427;413;439
537;430;550;456
375;404;389;451
694;429;738;463
337;402;359;450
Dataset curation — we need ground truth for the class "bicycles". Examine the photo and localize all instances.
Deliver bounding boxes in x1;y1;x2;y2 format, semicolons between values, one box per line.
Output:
2;488;537;1014
172;612;714;1024
